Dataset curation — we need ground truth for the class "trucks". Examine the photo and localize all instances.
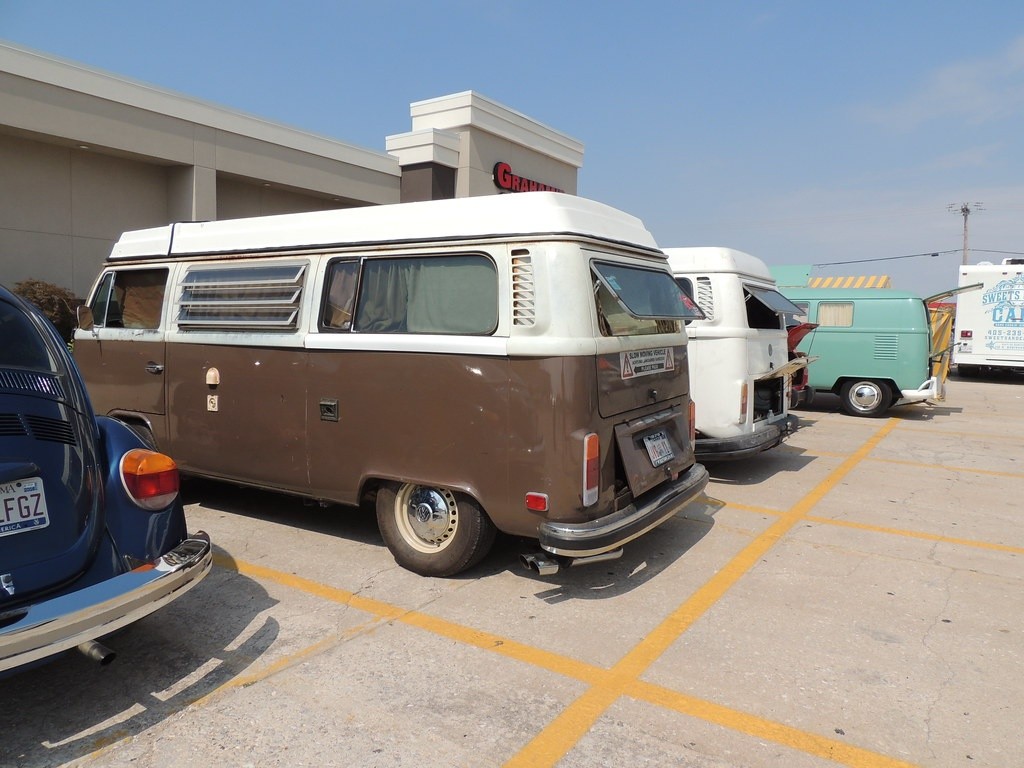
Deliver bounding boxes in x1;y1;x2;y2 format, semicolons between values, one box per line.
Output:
950;258;1024;379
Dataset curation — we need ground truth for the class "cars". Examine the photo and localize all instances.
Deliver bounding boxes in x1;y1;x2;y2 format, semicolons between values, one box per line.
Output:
1;284;212;674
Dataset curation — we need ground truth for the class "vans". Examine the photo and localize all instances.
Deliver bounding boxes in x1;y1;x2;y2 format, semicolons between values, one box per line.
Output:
768;262;983;420
70;192;711;579
601;248;806;463
